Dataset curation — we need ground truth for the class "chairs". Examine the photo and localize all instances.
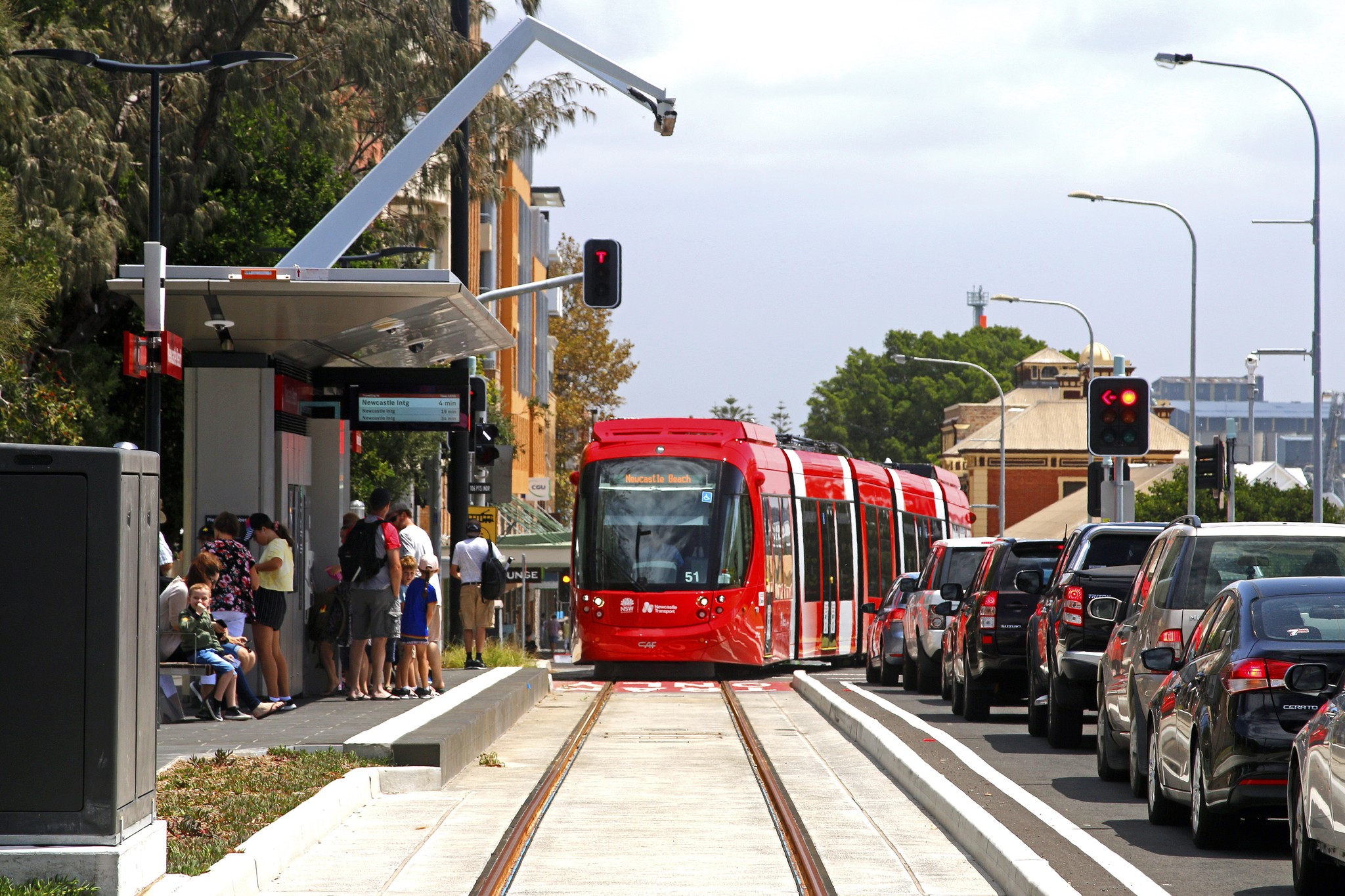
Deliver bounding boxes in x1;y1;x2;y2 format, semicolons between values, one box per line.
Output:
1261;602;1322;640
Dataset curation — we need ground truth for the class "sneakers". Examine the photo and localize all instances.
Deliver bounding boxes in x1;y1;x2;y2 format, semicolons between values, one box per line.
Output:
189;681;203;704
392;687;402;697
413;686;421;697
399;688;419;699
223;709;252;721
464;661;475;669
420;688;440;699
206;695;223;722
474;660;488;669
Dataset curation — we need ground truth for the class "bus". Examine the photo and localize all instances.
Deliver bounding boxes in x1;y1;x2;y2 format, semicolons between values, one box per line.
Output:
569;418;978;669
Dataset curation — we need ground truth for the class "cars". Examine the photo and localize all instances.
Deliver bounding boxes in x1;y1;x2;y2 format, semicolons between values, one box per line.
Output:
859;571;921;686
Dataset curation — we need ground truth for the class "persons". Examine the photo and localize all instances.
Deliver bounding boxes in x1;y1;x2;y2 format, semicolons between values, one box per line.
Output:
562;619;570;650
449;519;503;669
243;513;296;711
158;500;287;722
313;488;446;701
548;615;561;651
634;527;683;568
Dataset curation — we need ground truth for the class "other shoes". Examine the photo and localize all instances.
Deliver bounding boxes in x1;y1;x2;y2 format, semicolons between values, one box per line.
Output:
277;699;297;709
367;684;374;690
261;697;284;709
384;685;393;693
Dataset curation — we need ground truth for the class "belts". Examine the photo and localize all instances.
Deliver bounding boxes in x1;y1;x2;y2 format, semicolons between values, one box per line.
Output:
461;581;482;587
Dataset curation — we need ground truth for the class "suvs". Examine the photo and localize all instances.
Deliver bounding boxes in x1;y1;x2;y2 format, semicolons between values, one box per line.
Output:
1089;515;1345;896
934;538;1066;722
898;537;997;692
1012;520;1184;749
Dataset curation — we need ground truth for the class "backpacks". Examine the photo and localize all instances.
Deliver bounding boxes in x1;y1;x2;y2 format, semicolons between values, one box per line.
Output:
337;518;389;584
480;538;507;603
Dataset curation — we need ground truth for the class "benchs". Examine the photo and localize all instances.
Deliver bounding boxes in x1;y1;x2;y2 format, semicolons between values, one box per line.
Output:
158;631;216;721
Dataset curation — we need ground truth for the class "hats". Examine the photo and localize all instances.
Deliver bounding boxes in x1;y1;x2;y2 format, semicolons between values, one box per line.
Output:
419;554;439;571
243;513;269;542
158;499;168;523
387;502;408;516
195;525;214;541
465;519;481;532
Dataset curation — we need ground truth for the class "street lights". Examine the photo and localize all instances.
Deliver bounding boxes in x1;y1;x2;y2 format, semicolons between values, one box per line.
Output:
992;295;1095;518
1067;190;1198;521
890;353;1005;538
1152;51;1325;529
11;47;299;497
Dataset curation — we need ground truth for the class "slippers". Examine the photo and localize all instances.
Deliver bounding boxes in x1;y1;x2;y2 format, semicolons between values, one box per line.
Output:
256;701;287;720
435;688;446;693
372;694;400;700
346;690;370;701
316;689;342;697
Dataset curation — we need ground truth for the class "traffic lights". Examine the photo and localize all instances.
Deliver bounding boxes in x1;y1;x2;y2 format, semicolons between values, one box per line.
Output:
557;567;572;603
458;375;487;412
471;423;500;468
1191;437;1225;494
582;239;620;310
1086;375;1151;458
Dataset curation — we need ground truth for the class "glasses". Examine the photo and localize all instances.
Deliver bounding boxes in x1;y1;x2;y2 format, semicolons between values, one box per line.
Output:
387;513;402;523
206;576;217;586
